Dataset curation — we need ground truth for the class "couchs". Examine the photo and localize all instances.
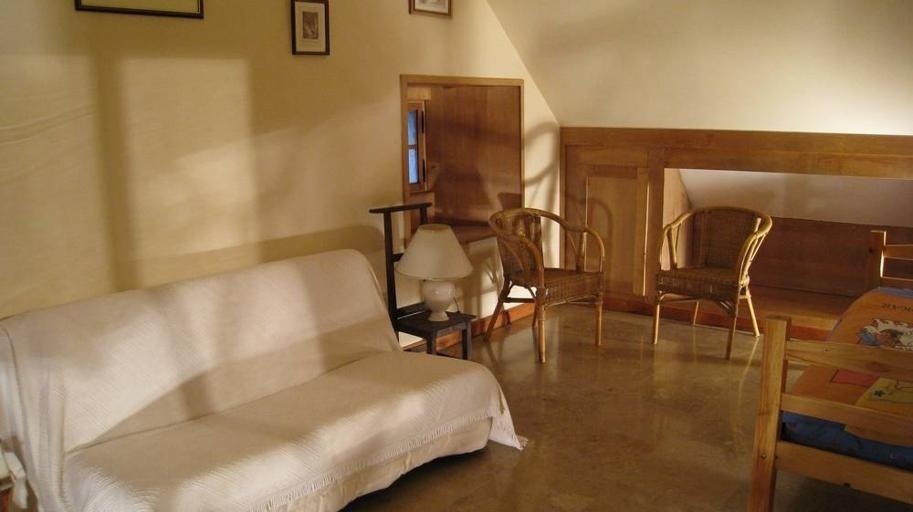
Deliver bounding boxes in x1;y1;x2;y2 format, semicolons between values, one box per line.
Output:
0;247;529;511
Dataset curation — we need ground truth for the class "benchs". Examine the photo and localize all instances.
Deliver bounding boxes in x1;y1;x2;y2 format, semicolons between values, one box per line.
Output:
746;230;912;512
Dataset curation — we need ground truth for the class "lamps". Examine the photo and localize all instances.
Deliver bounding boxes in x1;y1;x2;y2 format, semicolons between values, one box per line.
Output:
392;222;472;322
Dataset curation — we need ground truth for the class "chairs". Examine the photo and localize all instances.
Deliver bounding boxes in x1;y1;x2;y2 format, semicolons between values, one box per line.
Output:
483;206;607;365
367;201;477;361
650;204;773;360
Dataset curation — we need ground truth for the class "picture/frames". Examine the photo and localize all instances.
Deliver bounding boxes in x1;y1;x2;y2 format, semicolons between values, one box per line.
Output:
289;0;330;55
74;0;205;19
408;0;452;19
